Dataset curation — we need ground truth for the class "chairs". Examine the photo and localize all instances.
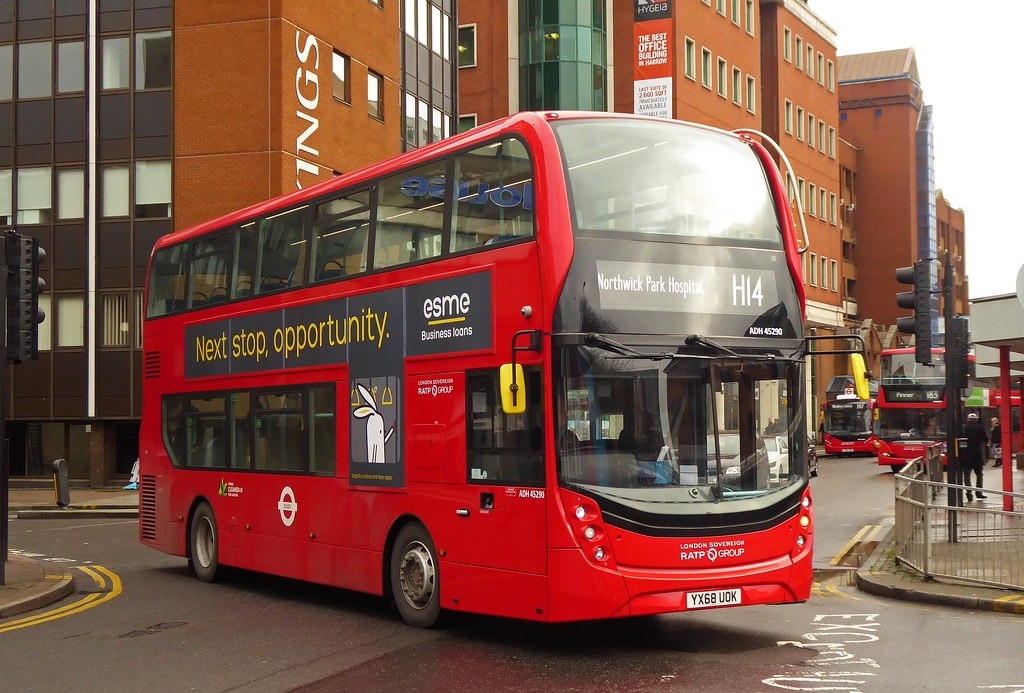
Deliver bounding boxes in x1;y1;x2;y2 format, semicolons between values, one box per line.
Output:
184;260;349;309
179;412;336;473
472;426;672;489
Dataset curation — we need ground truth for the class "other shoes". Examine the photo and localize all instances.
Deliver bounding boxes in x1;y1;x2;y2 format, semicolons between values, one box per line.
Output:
976;492;987;499
992;462;1001;467
966;494;973;501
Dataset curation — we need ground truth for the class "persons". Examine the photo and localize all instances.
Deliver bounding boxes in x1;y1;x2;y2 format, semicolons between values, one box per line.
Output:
764;419;785;435
990;417;1002;467
129;458;140;485
962;413;989;502
818;418;824;445
645;379;694;474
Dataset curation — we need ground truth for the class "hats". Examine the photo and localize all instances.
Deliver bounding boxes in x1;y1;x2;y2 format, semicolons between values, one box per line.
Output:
968;413;981;419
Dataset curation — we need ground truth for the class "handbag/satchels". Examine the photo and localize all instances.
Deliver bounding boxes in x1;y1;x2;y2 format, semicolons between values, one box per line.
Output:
992;443;1003;458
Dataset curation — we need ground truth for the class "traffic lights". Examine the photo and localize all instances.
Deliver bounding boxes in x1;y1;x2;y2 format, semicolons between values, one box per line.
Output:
6;232;47;365
895;260;940;369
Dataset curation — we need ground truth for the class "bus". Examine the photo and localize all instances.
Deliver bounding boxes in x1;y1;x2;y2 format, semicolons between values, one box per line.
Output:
995;389;1023;456
821;397;881;459
136;108;880;629
878;346;996;474
